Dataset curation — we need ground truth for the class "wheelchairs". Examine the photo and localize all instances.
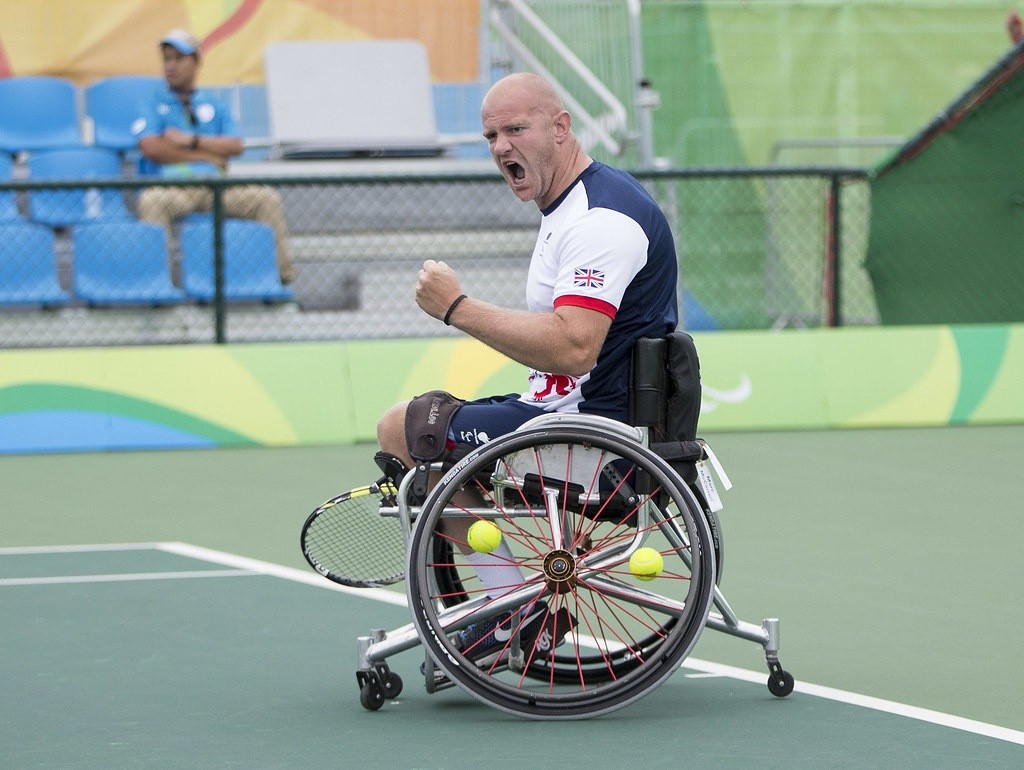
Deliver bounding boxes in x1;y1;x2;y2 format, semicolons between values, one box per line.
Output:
357;333;795;720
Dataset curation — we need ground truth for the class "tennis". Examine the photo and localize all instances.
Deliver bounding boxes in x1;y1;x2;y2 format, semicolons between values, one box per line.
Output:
465;519;504;555
628;546;665;583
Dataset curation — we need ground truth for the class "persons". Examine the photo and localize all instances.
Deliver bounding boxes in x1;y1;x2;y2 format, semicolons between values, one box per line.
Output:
134;30;300;289
377;72;680;680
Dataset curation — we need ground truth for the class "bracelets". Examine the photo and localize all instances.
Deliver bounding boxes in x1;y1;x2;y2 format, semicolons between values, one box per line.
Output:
191;135;200;150
443;294;468;326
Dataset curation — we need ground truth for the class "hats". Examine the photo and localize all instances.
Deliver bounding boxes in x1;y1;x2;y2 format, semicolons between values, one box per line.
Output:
159;29;199;55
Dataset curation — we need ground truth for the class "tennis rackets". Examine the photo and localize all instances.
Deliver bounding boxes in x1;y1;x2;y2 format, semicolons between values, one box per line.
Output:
298;483;415;590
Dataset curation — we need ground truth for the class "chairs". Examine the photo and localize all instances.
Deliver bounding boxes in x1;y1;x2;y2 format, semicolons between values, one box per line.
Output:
0;77;494;302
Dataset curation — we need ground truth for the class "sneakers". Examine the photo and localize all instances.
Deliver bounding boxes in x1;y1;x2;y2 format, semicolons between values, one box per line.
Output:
419;595;567;676
